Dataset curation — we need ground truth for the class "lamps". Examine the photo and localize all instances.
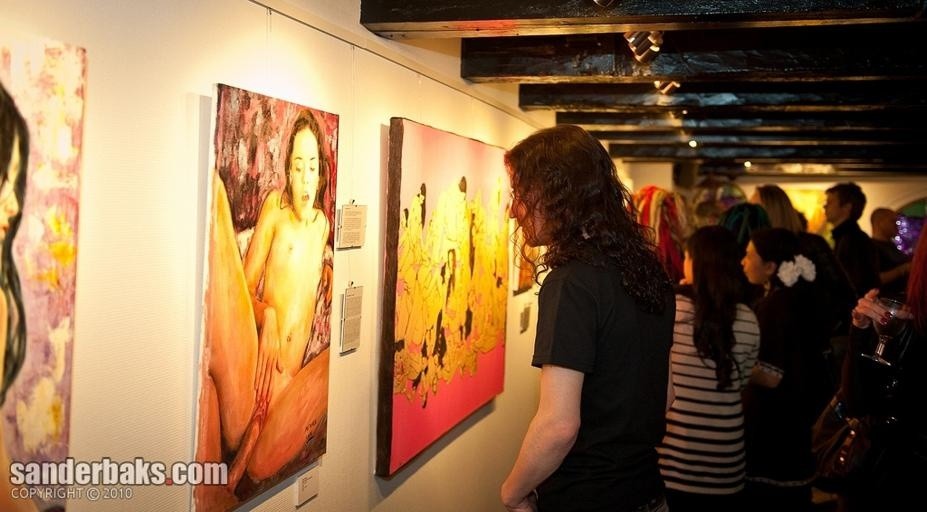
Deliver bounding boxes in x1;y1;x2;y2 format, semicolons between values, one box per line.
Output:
593;0;691;119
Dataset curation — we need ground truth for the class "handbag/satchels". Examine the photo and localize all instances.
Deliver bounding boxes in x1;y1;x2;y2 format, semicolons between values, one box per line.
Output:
809;383;884;492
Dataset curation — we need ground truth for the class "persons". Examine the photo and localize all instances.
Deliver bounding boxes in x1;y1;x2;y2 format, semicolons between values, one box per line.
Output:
1;86;42;510
500;125;674;509
197;115;512;510
658;181;927;512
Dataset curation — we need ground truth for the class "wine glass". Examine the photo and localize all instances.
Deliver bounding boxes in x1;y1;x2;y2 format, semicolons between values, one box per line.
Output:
860;296;912;367
